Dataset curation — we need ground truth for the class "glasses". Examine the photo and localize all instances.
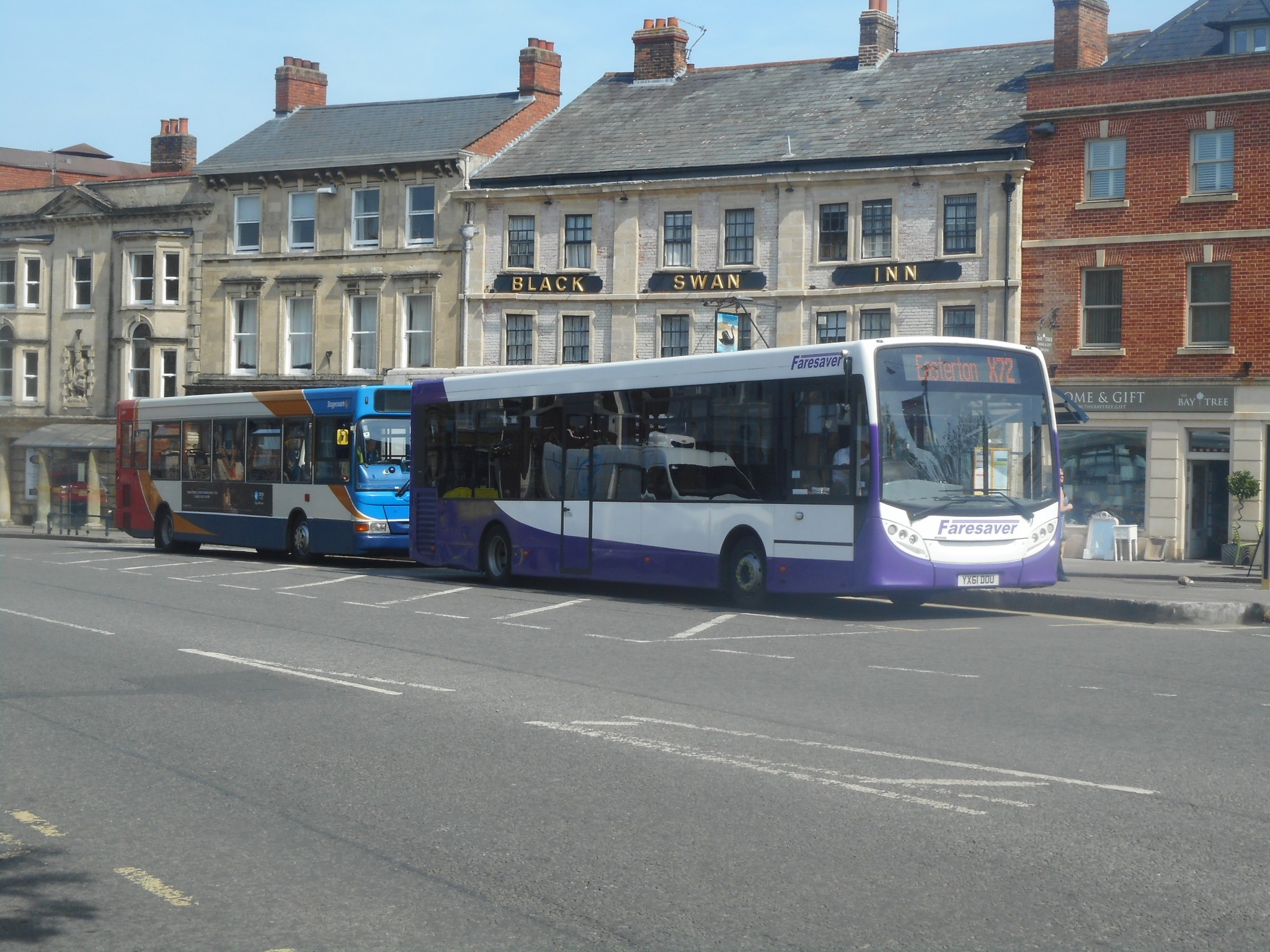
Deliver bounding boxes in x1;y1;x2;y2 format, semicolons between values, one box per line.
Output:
1060;474;1064;477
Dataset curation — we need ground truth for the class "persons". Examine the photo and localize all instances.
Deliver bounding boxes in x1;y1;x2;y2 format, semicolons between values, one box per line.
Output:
349;433;381;468
1058;468;1073;556
71;350;87;399
833;419;874;497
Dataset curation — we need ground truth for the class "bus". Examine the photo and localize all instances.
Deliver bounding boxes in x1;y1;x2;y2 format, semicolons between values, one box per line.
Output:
410;336;1093;603
114;382;419;565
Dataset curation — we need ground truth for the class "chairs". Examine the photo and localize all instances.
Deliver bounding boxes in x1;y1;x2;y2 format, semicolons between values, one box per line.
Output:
1232;526;1260;567
1256;521;1263;539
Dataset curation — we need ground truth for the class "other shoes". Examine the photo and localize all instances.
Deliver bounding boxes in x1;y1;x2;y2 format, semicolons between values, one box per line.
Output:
1057;575;1070;582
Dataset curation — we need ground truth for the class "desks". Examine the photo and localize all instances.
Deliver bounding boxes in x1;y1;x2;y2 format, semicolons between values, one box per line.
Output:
1112;524;1138;561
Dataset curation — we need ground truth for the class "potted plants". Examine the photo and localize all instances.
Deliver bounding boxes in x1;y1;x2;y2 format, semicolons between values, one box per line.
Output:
1221;470;1262;565
